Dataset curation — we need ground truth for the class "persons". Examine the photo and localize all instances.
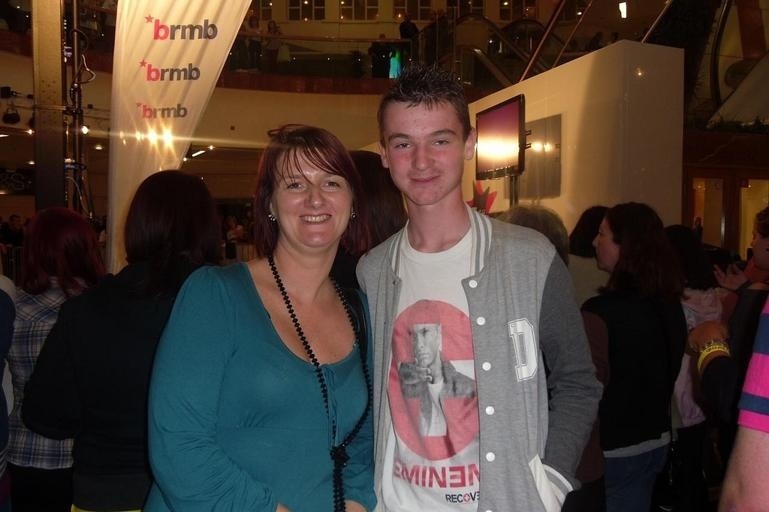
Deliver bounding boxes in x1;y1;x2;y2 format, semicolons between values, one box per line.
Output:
492;199;769;512
21;169;230;512
143;121;377;511
65;0;119;72
0;204;251;512
232;7;293;74
399;14;419;65
368;33;397;79
353;77;606;511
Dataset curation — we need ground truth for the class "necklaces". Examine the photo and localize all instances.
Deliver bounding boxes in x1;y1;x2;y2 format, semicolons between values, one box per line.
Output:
269;252;376;511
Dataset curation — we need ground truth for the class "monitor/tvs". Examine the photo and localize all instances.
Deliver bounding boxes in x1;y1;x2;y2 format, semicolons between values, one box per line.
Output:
475;93;526;181
